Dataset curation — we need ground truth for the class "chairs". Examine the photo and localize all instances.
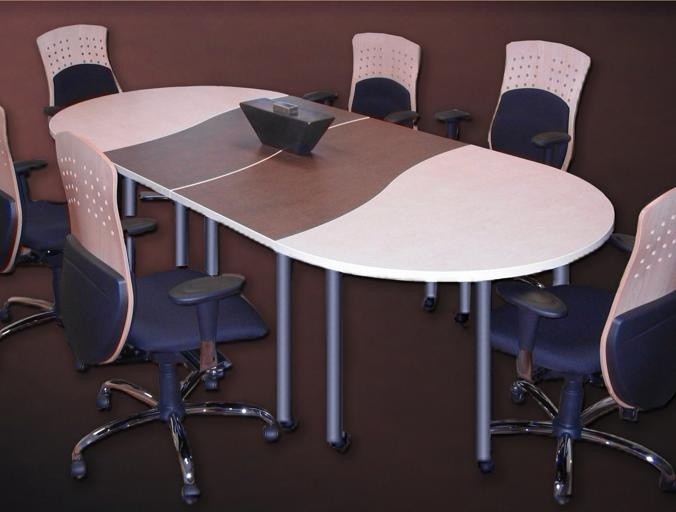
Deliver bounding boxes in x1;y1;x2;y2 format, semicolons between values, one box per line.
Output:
488;187;675;512
33;23;123;121
1;107;157;376
55;128;281;506
303;32;422;132
421;40;593;325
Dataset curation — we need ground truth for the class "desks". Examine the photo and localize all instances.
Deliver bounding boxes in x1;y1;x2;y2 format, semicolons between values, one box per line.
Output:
47;83;616;474
